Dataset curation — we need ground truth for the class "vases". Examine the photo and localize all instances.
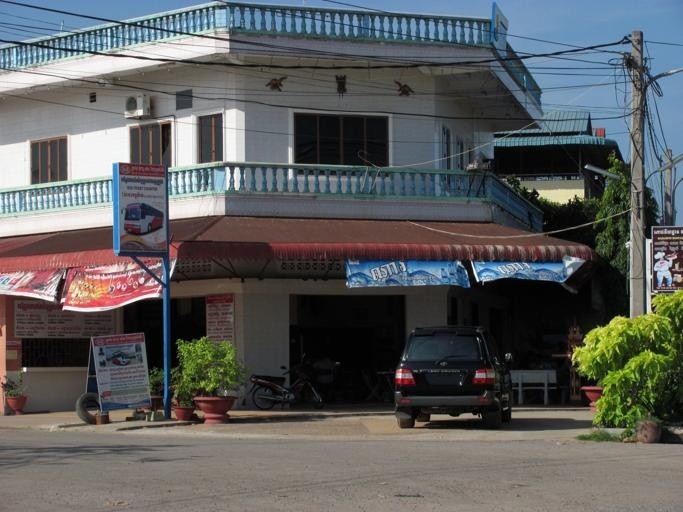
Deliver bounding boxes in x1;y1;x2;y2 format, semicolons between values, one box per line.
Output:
7;397;27;413
581;386;604;407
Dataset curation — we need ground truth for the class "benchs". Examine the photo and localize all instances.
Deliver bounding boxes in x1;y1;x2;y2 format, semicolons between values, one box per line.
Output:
507;369;558;406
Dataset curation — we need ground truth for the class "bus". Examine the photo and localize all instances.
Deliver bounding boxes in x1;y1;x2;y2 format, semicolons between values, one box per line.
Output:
120;203;163;235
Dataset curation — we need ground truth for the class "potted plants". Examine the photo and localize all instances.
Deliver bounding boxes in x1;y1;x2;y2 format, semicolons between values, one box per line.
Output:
635;412;664;444
149;335;247;424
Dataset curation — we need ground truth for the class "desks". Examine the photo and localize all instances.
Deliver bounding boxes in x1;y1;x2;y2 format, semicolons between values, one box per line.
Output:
376;370;396;404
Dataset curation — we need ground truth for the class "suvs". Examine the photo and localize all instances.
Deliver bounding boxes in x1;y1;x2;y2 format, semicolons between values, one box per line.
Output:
393;327;510;430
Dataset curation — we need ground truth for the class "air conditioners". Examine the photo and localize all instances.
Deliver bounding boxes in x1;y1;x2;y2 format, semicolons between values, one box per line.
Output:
123;93;149;118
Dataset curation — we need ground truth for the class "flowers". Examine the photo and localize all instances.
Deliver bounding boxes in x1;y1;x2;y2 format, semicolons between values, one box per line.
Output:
0;367;28;397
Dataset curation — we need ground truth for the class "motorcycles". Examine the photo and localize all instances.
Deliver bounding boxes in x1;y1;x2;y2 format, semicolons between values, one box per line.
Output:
246;354;326;409
